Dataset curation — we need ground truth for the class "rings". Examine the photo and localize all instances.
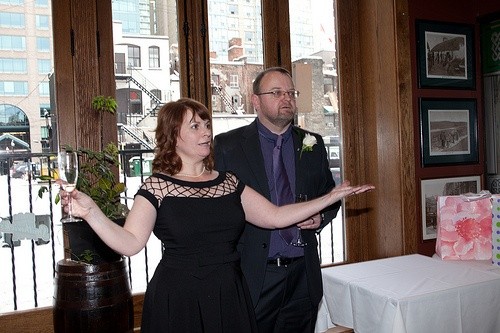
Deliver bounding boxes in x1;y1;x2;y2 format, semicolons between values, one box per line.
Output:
310;217;315;224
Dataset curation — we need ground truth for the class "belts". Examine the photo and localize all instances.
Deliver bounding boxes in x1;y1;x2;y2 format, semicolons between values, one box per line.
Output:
267;256;305;266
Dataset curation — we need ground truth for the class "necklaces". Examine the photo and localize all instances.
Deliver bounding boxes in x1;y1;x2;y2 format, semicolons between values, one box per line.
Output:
176;164;206;178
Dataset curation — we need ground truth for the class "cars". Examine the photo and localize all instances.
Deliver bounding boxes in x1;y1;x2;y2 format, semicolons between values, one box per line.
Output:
324;136;341;169
9;155;60;183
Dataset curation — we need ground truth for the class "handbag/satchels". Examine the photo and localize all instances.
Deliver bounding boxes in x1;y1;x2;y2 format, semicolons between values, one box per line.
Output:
435;190;492;259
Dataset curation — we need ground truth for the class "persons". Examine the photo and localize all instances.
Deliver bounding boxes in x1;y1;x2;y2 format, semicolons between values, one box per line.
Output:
212;66;341;333
59;98;376;333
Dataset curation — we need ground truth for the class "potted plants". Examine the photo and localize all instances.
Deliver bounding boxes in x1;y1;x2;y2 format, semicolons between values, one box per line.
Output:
33;94;130;264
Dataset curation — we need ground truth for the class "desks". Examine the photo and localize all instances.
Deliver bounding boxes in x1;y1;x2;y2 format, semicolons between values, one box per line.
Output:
314;253;500;333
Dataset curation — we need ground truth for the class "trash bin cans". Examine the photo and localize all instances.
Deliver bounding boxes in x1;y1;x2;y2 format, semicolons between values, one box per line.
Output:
129;162;136;177
134;160;140;176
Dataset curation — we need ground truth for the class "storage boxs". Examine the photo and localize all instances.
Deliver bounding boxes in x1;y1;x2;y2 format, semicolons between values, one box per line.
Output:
435;194;500;266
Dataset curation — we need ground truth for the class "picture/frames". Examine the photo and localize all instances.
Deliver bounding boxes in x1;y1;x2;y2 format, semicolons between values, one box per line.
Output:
417;96;479;167
419;173;484;244
413;17;478;91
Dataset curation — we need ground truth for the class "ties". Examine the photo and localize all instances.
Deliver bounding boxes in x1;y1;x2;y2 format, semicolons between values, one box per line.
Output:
272;136;299;245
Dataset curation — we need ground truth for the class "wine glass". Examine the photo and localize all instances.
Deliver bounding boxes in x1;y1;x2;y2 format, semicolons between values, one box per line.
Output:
57;151;83;223
290;193;308;247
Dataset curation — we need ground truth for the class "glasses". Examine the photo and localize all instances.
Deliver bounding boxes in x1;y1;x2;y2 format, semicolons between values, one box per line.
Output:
256;89;300;99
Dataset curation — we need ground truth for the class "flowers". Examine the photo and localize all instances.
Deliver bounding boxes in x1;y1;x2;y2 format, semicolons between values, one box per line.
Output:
300;133;318;159
